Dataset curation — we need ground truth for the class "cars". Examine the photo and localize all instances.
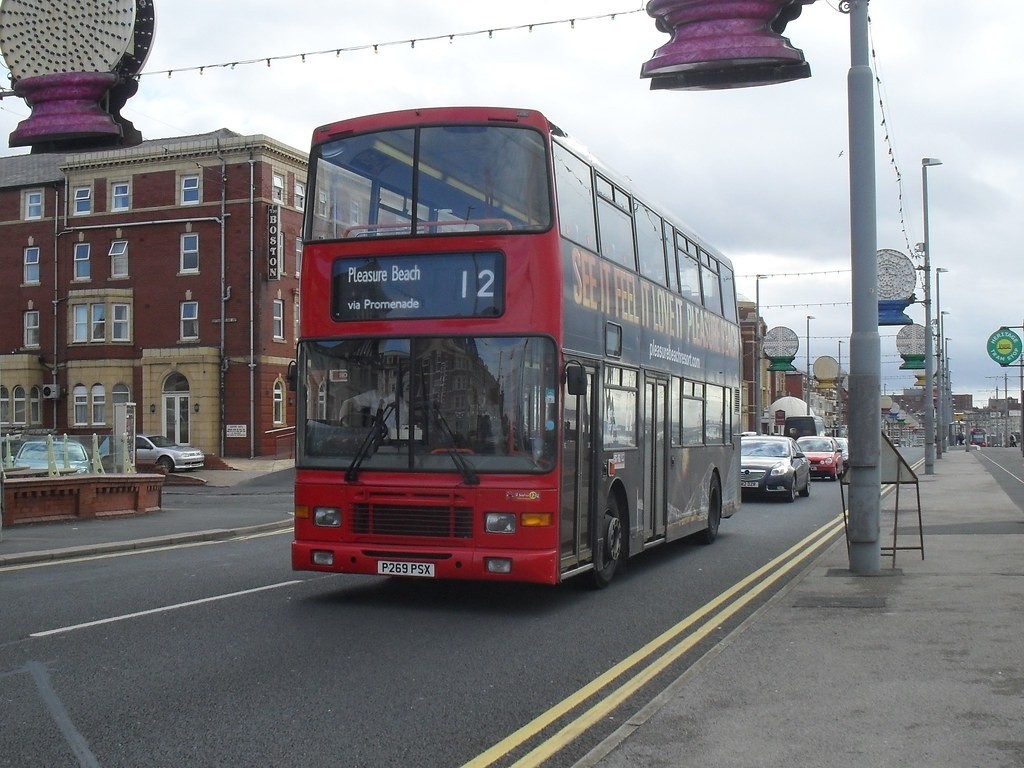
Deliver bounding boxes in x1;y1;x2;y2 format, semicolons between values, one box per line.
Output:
796;436;844;481
830;436;849;468
97;432;205;475
740;435;811;504
12;440;92;478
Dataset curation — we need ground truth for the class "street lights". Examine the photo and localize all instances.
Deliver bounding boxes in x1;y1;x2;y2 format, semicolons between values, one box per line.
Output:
806;316;816;415
941;310;950;455
934;267;949;460
944;337;952;447
757;274;768;435
837;341;846;438
921;157;945;474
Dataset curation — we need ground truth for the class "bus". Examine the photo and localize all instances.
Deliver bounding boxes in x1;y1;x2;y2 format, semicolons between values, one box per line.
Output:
284;106;743;589
971;428;987;448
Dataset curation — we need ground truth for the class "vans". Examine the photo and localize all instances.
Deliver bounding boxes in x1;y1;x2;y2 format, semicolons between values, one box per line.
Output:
784;415;829;441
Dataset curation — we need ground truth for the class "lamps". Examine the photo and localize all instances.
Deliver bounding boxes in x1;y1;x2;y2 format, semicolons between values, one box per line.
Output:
151;404;156;413
194;403;200;412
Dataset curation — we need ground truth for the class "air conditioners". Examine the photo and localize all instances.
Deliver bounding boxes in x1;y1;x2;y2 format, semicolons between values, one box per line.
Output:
43;383;60;399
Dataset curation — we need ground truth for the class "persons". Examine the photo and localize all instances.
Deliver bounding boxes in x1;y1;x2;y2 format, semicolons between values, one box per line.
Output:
1010;435;1018;447
339;369;409;439
